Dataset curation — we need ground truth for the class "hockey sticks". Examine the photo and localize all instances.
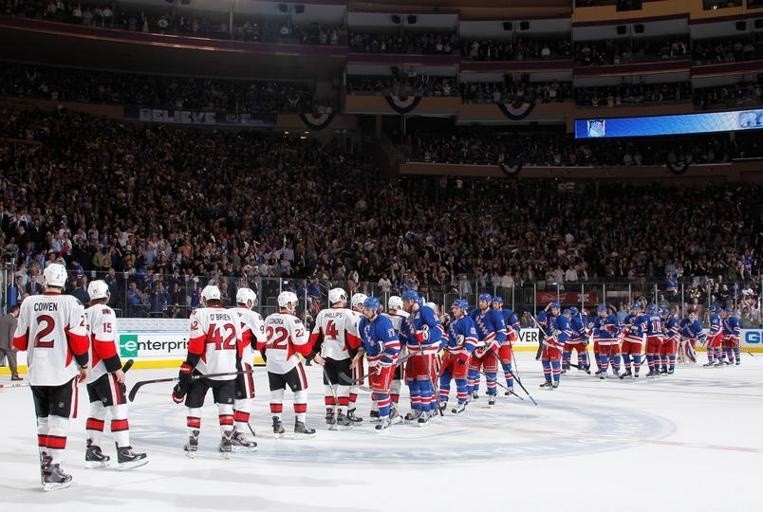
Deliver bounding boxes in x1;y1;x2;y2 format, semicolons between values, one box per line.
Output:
527;311;582;370
129;370;254;402
337;345;433;384
407;308;445;416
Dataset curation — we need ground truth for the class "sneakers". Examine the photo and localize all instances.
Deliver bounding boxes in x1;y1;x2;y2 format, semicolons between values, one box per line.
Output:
661;368;667;376
504;389;515;397
584;367;591;375
337;413;354;429
437;401;447;411
404;405;419;422
325;413;336;430
561;368;567;376
715;361;723;366
653;369;660;376
729;358;734;366
735;359;741;367
614;371;621;380
390;407;402;423
488;395;496;408
430;409;437;417
420;412;431;427
622;369;632;378
473;391;480;400
374;418;390;434
232;432;257;453
347;410;363;425
704;361;713;367
452;403;465;416
646;369;653;377
540;381;551;389
595;369;600;377
634;369;640;377
600;371;608;379
552;381;559;388
370;409;379;423
668;368;675;376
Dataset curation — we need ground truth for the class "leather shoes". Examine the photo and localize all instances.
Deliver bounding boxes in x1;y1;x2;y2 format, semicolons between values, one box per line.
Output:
11;377;24;381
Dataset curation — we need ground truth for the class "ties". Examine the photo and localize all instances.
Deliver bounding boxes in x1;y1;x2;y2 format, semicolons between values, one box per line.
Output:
31;281;35;292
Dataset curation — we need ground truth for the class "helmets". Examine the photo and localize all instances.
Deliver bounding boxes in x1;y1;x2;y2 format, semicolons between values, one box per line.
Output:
550;303;561;308
43;263;68;287
364;297;380;310
402;290;420;303
277;291;298;308
631;304;641;308
452;299;469;311
426;302;436;313
328;288;346;304
388;296;403;310
597;304;608;312
647;305;655;311
351;293;367;307
478;292;491;302
726;307;733;313
569;306;579;314
492;296;504;303
87;280;108;301
660;306;669;314
200;286;220;303
710;304;719;313
235;288;256;303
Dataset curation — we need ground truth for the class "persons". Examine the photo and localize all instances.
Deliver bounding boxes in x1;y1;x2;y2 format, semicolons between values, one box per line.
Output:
0;0;762;110
172;286;242;459
0;110;763;319
14;263;89;493
229;283;742;435
84;280;146;469
0;304;22;381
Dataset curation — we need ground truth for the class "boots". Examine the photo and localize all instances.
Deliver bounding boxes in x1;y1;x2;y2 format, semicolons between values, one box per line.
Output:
273;416;285;439
41;464;72;494
84;448;110;469
294;418;316;439
218;432;233;459
117;447;148;472
184;436;198;459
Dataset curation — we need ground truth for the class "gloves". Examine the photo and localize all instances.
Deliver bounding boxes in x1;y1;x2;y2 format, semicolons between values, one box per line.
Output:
544;337;555;345
607;325;614;333
178;366;193;387
489;342;499;353
622;328;629;338
707;334;712;341
456;353;469;365
506;331;517;342
728;334;735;343
414;331;426;342
375;364;389;376
